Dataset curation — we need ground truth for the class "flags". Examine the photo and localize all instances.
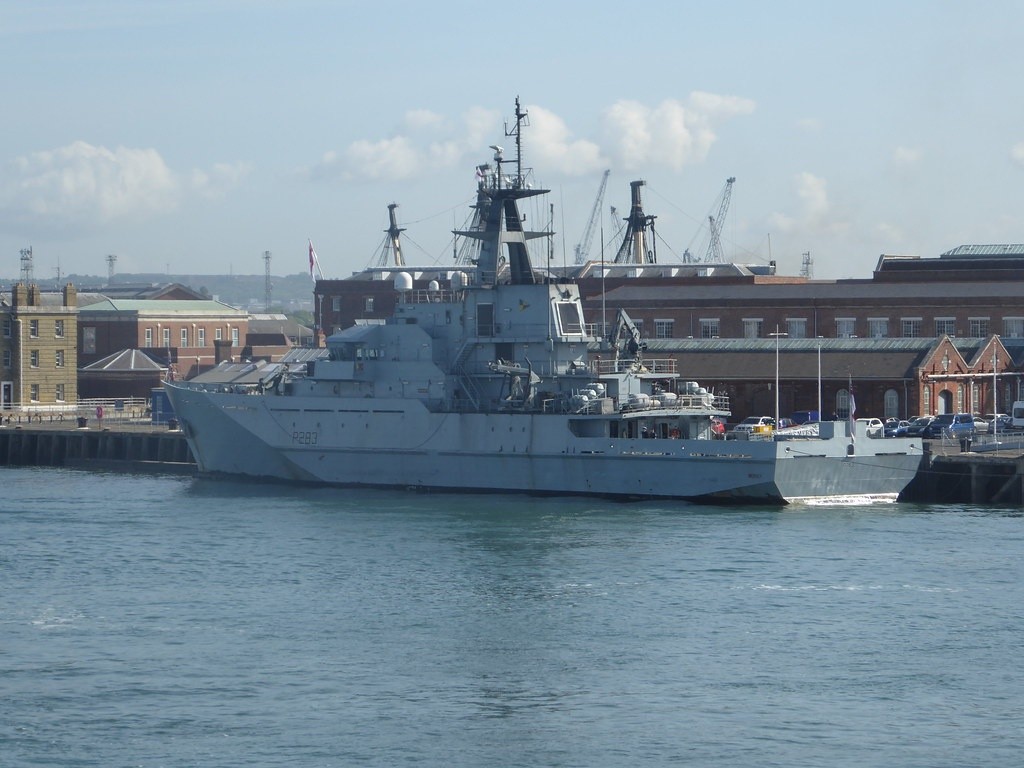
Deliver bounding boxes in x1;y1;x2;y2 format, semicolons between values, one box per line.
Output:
849;380;856;443
307;239;317;282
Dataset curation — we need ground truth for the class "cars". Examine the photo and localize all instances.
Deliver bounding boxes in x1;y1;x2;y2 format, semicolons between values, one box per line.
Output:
733;410;817;432
711;419;724;435
856;401;1024;439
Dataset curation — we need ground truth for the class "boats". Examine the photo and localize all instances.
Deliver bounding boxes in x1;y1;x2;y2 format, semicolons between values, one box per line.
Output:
162;95;923;507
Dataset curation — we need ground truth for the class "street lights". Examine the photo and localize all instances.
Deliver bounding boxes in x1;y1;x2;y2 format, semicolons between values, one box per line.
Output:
815;336;824;423
768;324;789;432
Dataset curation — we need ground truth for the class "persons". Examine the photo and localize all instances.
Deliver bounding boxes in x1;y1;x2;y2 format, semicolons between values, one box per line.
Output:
257;378;265;396
670;426;679;440
829;409;838;421
643;426;656;439
711;421;725;435
